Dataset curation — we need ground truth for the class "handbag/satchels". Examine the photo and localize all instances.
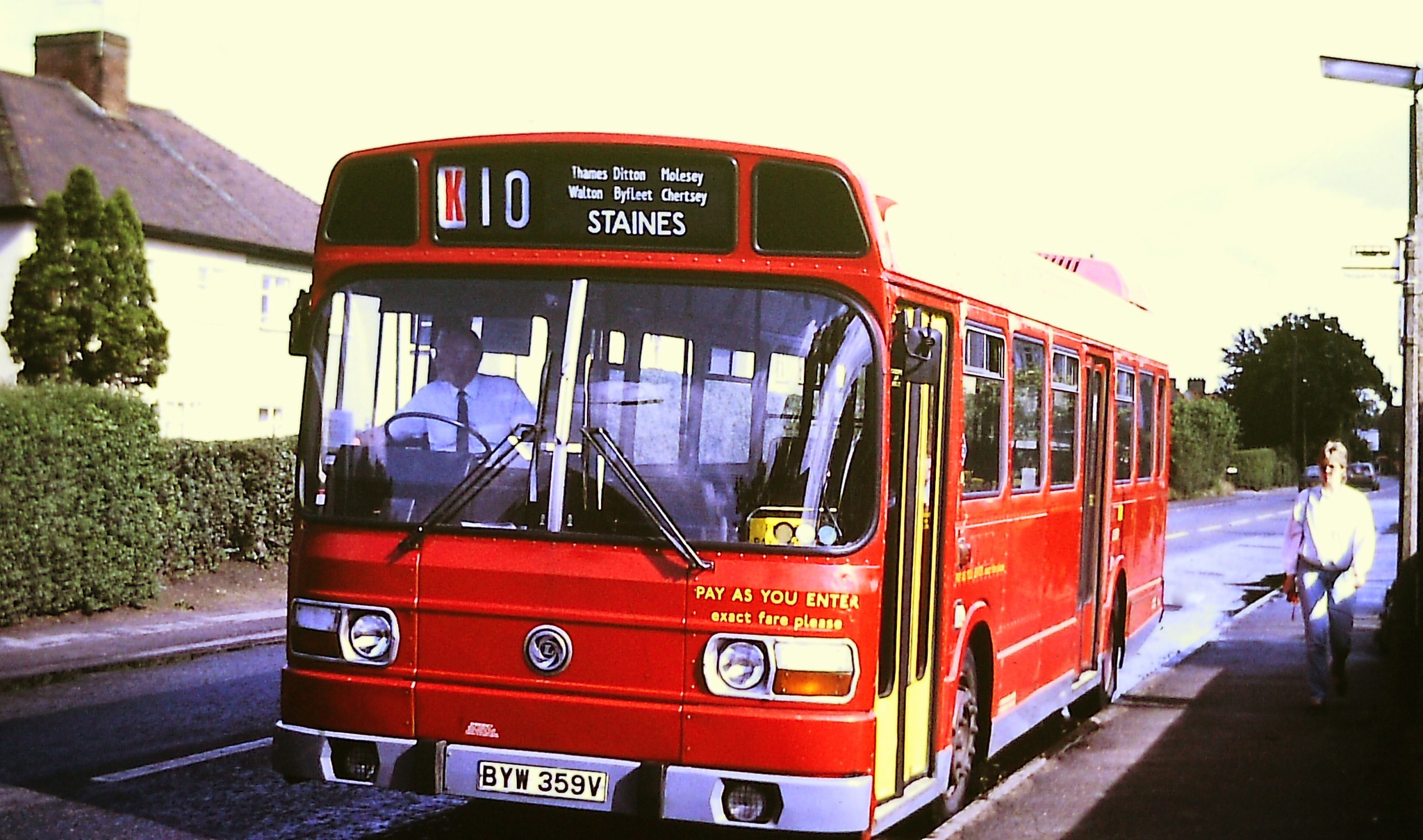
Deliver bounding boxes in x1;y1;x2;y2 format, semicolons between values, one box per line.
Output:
1283;572;1301;604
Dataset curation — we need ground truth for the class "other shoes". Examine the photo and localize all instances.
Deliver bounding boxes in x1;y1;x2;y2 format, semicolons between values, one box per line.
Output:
1306;684;1327;708
1336;681;1358;702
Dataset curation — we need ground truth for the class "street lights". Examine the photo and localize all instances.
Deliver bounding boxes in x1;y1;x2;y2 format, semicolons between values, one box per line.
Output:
1321;50;1421;564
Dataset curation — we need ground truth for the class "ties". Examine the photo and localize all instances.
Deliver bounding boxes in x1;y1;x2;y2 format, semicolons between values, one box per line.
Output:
456;392;469;455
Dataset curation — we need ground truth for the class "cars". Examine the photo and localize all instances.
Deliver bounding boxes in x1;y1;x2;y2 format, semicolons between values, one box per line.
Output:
1298;465;1322;492
1345;460;1380;492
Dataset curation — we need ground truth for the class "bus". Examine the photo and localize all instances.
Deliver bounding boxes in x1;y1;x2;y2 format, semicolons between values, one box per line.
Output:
270;130;1187;840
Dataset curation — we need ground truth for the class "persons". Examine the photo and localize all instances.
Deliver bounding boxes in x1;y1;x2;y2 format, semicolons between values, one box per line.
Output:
355;324;537;468
1283;442;1376;704
